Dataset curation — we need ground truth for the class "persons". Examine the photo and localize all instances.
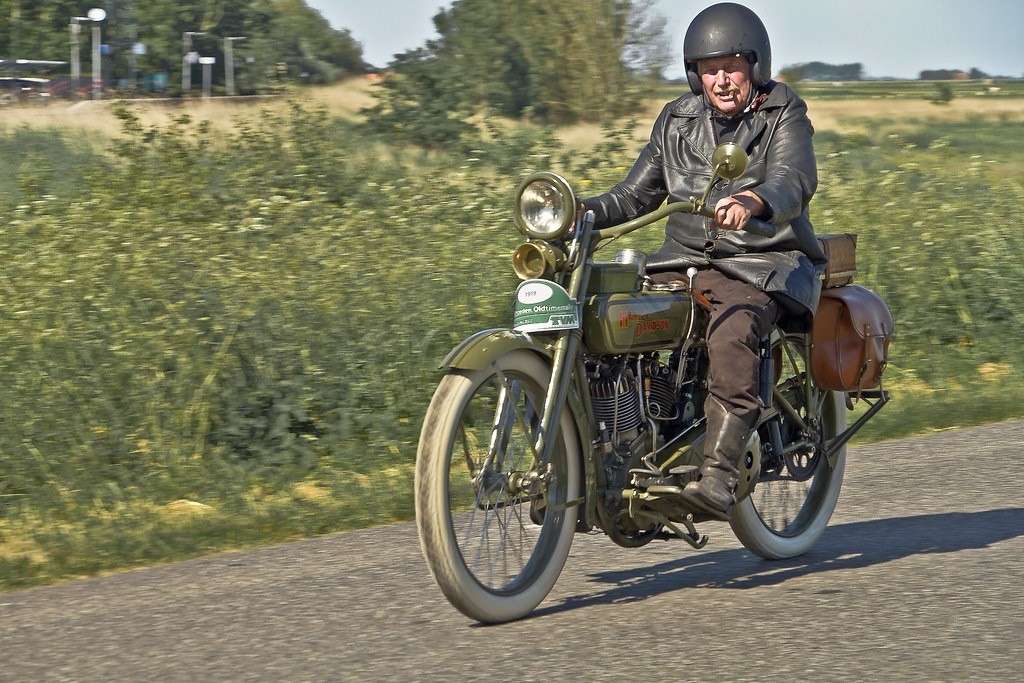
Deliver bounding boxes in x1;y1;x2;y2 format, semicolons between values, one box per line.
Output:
573;2;826;514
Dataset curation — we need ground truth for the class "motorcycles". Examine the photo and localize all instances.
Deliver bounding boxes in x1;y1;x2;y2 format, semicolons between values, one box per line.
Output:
410;142;896;626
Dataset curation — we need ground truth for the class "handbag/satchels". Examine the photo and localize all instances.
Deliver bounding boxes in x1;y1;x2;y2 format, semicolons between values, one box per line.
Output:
810;284;894;403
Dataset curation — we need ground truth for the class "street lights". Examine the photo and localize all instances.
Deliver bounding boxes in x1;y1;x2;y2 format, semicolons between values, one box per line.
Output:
181;30;207;94
224;35;248;97
66;17;93;79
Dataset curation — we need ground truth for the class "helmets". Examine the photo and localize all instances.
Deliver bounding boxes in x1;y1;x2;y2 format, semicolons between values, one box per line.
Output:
683;2;772;96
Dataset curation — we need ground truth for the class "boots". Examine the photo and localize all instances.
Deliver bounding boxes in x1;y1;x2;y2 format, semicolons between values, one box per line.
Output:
684;392;762;512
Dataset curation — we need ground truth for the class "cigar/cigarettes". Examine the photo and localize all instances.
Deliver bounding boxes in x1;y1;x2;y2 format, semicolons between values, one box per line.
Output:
729;90;735;100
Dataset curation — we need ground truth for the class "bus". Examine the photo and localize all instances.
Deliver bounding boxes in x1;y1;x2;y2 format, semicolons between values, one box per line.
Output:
0;57;70;105
